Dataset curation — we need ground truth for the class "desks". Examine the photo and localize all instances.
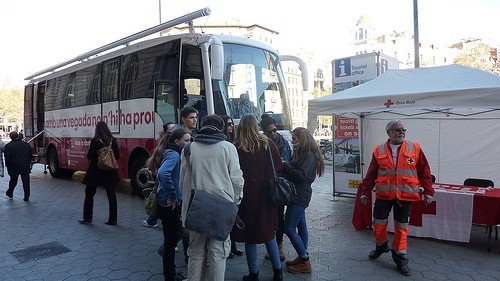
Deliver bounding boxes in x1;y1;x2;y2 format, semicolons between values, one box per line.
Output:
371;183;500;244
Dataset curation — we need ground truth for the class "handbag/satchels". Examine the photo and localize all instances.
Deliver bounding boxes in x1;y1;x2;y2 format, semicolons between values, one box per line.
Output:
96;138;120;169
145;189;158;216
184;189;238;242
267;140;298;206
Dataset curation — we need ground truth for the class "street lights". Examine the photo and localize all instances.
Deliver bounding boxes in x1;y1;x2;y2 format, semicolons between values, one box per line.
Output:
315;78;324;144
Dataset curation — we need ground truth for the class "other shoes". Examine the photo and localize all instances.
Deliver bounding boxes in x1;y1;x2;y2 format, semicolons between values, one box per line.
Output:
175;274;188;281
267;267;283;280
392;254;411;277
143;220;158;227
284;257;311;274
78;218;92;223
23;198;29;201
227;248;244;259
263;240;284;261
6;191;13;198
156;251;163;263
368;244;392;261
104;221;109;225
243;270;260;281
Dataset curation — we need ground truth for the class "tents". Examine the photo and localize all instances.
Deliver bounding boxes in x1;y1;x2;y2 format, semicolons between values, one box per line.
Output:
307;64;500;136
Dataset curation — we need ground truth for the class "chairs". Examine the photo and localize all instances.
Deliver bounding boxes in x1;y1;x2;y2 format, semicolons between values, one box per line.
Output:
463;178;494;234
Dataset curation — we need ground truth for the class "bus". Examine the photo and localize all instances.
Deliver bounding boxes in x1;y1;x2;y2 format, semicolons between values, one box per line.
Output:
24;8;308;201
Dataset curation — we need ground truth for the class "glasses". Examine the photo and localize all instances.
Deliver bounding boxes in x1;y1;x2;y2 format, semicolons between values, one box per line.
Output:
394;128;406;132
227;122;232;126
265;128;277;135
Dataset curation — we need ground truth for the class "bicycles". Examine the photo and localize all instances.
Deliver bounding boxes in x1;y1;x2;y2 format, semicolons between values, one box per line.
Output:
318;142;332;159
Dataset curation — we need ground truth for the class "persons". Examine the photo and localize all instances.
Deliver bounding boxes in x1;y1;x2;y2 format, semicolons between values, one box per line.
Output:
149;127;190;281
221;115;235;143
78;121;119;225
282;127;324;274
179;114;243;281
180;107;198;137
232;114;283;281
0;134;5;178
144;122;176;228
235;94;259;118
4;131;32;201
19;130;24;140
361;119;434;276
260;117;291;260
